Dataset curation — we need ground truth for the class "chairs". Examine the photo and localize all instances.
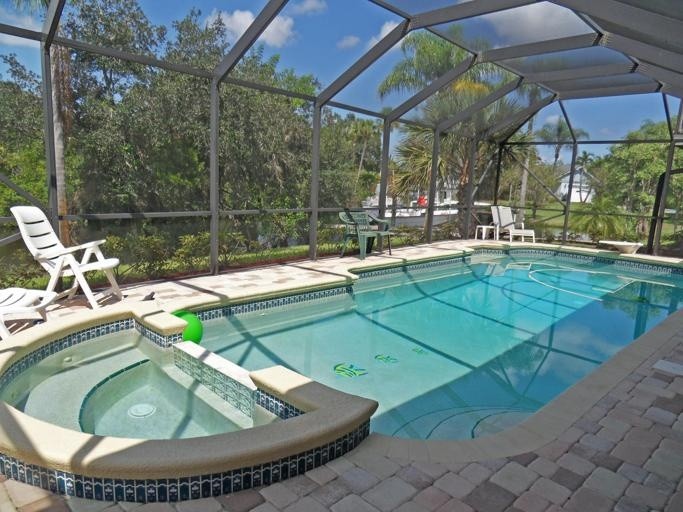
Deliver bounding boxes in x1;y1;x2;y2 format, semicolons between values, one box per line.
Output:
339;209;391;260
489;205;536;242
8;205;123;311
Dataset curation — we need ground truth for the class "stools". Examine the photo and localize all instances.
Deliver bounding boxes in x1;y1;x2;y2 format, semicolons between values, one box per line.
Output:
474;224;497;240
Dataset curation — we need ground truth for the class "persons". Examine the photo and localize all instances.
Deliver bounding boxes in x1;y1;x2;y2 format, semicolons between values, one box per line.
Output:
417;195;429;209
